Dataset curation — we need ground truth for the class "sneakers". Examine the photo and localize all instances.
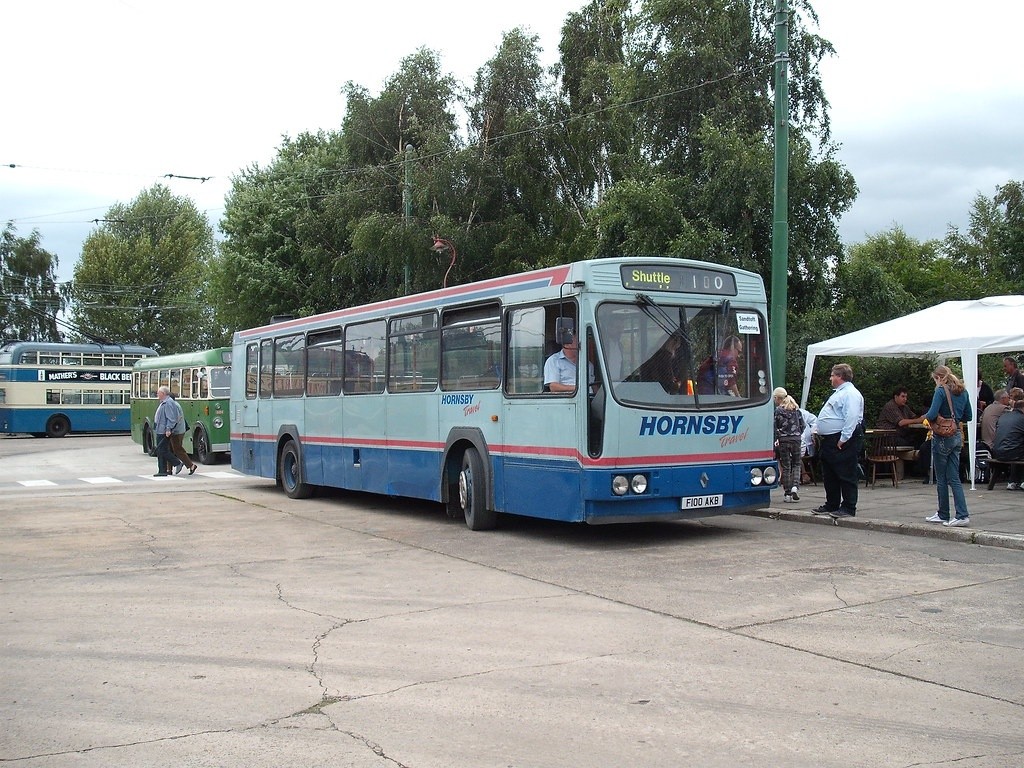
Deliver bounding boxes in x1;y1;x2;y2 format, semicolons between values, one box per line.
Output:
1007;482;1020;489
1020;482;1024;488
922;478;934;484
784;495;792;502
829;509;852;517
925;512;950;522
791;486;800;500
811;506;831;514
943;517;970;527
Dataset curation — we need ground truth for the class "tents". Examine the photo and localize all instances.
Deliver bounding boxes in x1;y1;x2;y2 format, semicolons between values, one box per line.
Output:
800;295;1024;491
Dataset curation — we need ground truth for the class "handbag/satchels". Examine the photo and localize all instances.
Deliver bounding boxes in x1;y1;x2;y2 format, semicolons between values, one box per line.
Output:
184;420;190;431
930;413;957;437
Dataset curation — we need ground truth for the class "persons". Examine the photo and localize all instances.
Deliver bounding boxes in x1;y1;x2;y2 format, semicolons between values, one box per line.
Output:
596;314;682;395
773;387;818;503
153;386;197;476
925;364;972;526
697;335;742;397
544;329;595;393
876;356;1024;491
811;364;864;518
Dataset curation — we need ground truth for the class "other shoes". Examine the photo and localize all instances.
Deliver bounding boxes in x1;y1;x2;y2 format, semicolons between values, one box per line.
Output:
153;472;167;476
188;465;197;475
176;462;183;474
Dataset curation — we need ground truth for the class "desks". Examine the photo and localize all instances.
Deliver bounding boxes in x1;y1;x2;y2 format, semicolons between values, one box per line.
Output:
859;428;888;484
910;423;983;484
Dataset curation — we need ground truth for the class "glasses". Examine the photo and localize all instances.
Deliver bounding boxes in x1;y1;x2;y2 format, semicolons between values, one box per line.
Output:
735;346;742;354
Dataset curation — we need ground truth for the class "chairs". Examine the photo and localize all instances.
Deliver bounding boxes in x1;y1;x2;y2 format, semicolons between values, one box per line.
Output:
865;429;899;491
975;441;994;482
248;365;484;395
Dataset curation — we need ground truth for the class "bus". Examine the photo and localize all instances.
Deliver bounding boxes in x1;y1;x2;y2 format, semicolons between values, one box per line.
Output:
129;346;233;465
0;342;160;438
229;256;777;531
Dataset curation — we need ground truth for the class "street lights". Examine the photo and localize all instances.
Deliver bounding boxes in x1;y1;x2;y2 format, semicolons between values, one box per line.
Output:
430;238;458;288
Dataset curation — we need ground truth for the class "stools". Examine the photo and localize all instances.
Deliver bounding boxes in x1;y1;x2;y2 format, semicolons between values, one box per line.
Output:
985;458;1024;491
799;456;817;487
885;446;915;480
